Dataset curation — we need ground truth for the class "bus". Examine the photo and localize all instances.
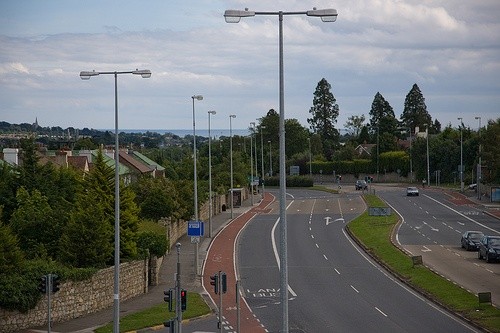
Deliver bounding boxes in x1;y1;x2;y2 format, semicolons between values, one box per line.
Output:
406;187;419;196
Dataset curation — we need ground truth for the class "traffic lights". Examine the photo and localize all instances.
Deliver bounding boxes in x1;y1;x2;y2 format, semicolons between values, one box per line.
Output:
164;319;174;332
38;275;48;294
210;272;227;294
52;276;60;295
163;289;173;312
178;289;187;313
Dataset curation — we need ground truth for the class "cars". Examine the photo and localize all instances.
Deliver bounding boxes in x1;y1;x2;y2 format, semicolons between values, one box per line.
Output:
354;180;369;190
460;230;500;264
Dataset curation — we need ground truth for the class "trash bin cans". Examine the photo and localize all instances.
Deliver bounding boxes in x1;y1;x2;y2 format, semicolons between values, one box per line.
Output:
222;205;226;211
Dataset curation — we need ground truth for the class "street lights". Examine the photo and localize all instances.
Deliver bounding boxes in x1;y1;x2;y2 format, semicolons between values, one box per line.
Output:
403;127;413;183
423;123;430;187
475;116;482;200
222;7;338;333
192;94;203;277
248;121;265;206
207;110;217;239
307;137;312;177
268;141;272;179
79;68;153;333
457;117;464;193
229;114;236;220
